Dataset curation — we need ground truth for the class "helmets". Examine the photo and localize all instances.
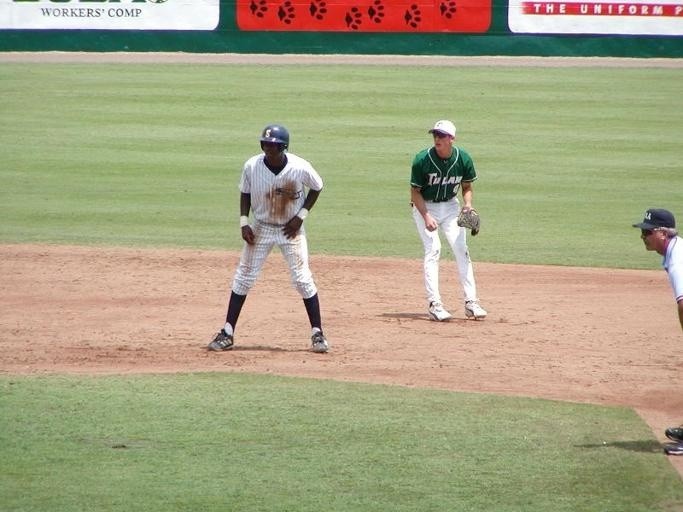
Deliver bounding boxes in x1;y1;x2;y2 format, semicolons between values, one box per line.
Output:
258;124;290;149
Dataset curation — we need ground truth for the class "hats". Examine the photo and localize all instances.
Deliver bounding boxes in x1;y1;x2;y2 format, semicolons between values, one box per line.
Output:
632;207;676;231
428;119;456;139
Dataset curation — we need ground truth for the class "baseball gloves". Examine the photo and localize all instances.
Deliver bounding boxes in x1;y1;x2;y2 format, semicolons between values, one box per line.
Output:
457;206;480;236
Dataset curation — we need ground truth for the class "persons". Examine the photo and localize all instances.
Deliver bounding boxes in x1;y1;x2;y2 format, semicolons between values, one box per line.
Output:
409;119;488;322
207;124;331;353
633;208;683;456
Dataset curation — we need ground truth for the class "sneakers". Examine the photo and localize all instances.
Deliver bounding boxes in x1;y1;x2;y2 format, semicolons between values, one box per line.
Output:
464;300;487;319
429;301;452;323
663;445;683;455
310;325;329;354
208;328;234;352
664;427;683;442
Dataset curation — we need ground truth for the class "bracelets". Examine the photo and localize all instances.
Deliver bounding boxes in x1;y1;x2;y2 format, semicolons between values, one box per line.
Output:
296;208;310;221
240;216;249;229
